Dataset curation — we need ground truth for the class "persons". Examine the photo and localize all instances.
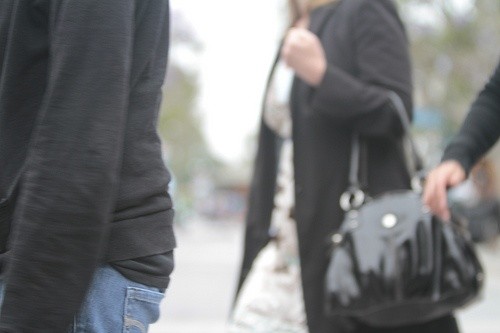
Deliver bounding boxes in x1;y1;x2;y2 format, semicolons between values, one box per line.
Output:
222;1;460;333
422;59;500;223
0;0;175;333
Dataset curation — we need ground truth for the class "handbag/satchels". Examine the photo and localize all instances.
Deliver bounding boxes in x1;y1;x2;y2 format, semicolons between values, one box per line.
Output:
322;86;484;329
